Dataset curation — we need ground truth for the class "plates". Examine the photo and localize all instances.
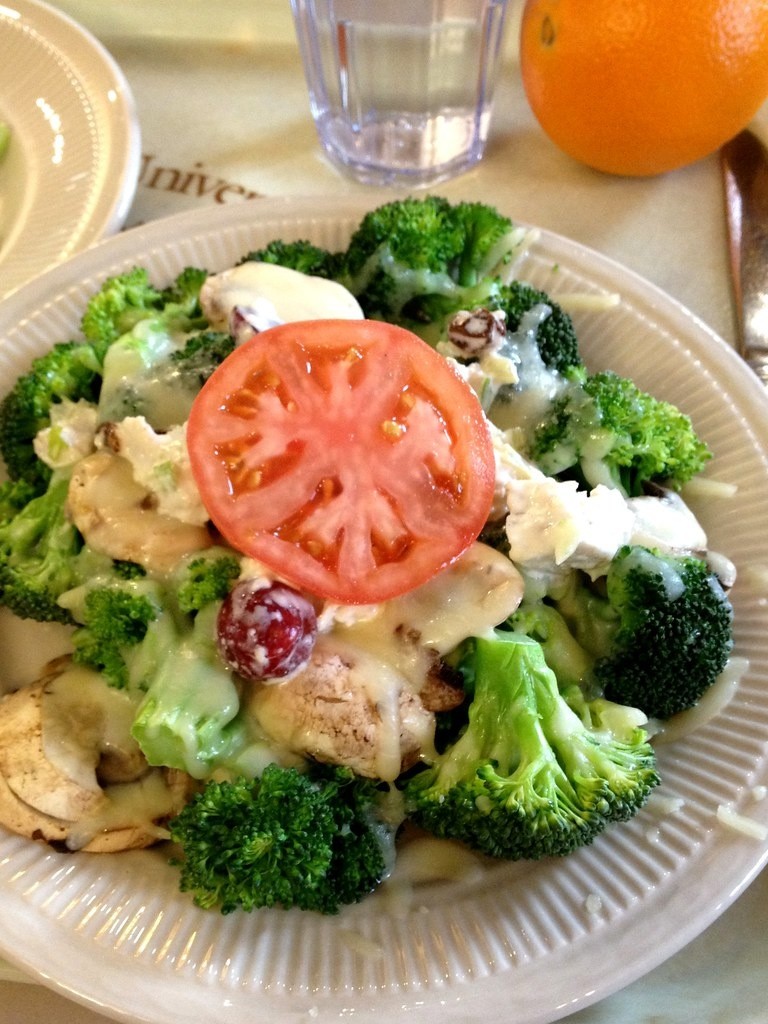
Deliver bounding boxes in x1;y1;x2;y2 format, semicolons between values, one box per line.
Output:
0;196;768;1024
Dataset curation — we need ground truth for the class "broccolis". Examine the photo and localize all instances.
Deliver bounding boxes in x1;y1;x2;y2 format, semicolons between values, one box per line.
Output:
0;196;729;908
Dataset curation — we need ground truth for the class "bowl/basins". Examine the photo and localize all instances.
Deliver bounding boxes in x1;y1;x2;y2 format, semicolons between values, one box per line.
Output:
0;0;142;303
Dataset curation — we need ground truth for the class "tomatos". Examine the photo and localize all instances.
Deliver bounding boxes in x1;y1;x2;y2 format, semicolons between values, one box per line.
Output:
189;321;492;602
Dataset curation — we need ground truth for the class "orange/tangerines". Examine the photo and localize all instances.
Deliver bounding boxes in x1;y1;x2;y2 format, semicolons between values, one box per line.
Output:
519;0;768;177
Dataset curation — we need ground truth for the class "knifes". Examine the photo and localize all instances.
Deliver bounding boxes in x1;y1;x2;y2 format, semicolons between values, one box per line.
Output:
721;128;768;388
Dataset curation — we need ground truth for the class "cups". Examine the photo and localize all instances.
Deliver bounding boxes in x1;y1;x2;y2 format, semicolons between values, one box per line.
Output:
289;0;508;190
